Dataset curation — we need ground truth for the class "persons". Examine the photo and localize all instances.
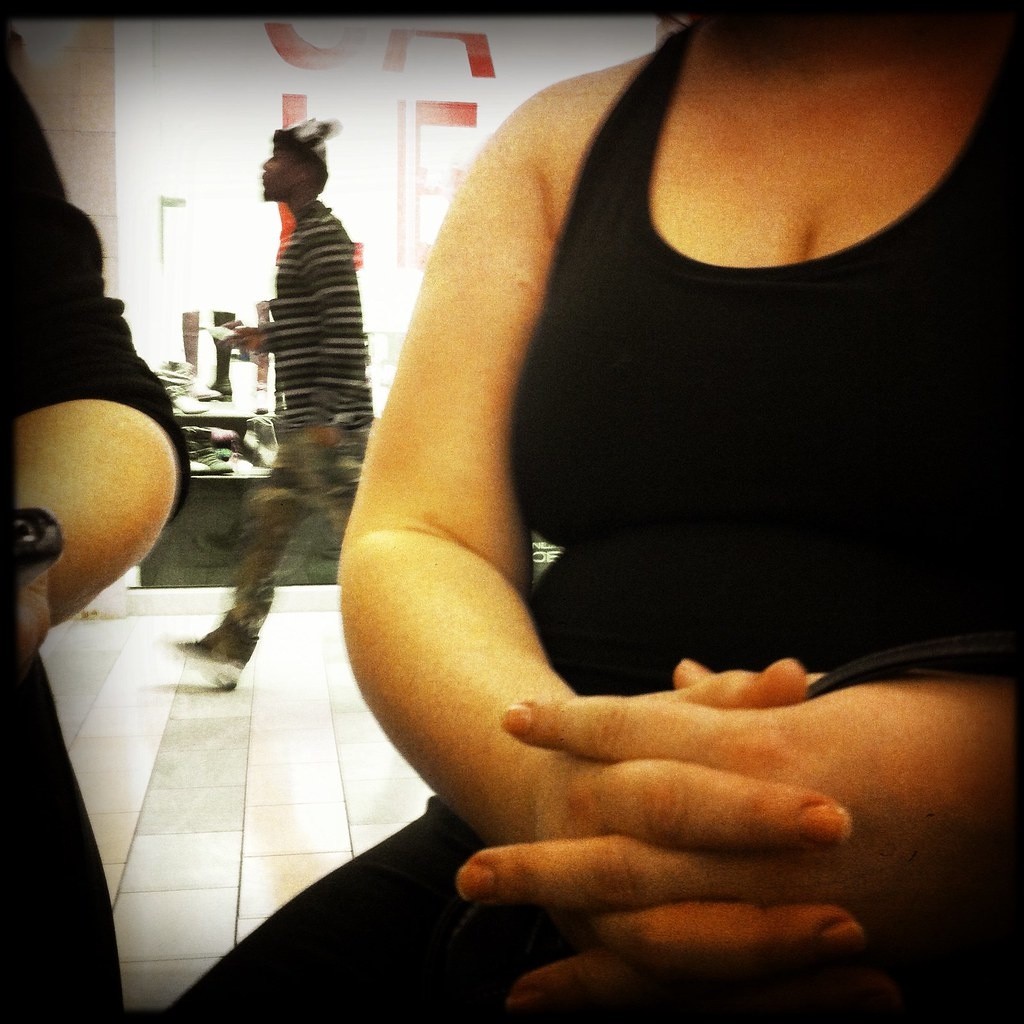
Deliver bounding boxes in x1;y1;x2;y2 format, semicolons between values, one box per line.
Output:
146;0;1024;1023
178;119;373;684
0;50;191;1024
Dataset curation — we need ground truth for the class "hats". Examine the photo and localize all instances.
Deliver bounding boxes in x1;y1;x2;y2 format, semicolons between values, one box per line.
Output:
272;119;334;184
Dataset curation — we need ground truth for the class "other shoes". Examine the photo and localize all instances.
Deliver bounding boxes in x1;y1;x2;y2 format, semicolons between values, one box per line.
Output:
165;638;241;689
